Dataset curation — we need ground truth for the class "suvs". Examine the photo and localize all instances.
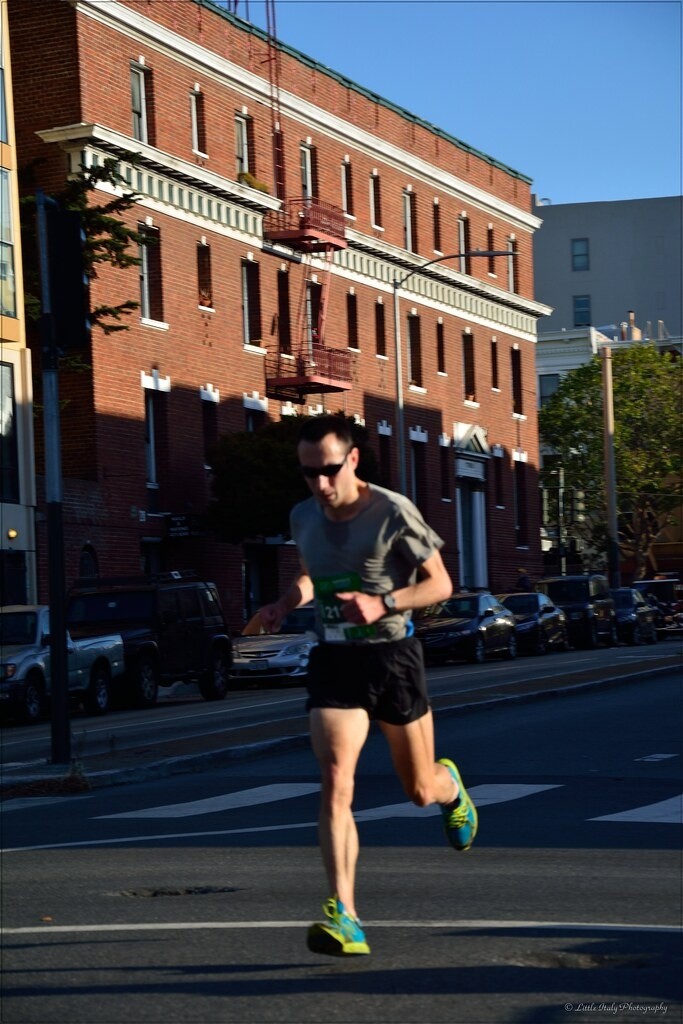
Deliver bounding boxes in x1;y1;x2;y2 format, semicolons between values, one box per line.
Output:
521;573;621;650
65;568;235;711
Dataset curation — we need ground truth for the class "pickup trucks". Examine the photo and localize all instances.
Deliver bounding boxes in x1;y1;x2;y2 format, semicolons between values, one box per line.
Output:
0;604;128;724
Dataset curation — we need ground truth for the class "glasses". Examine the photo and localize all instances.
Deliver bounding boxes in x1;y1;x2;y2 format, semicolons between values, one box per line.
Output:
299;443;354;479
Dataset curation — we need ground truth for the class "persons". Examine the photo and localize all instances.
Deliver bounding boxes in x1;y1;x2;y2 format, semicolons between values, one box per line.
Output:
640;565;650;579
259;411;478;957
512;569;532;592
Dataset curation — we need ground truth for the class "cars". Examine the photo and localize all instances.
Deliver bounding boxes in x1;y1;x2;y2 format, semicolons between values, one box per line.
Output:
492;594;573;655
411;590;521;664
630;577;682;642
611;585;660;648
228;605;319;692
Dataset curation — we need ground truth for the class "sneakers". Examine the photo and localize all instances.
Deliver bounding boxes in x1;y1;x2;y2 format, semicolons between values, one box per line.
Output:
307;893;369;956
437;758;478;852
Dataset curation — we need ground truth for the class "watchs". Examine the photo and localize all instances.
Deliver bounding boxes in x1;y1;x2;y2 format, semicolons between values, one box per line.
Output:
381;593;396;617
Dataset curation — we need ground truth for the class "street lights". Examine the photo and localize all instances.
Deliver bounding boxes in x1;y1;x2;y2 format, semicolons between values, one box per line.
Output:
392;250;520;496
